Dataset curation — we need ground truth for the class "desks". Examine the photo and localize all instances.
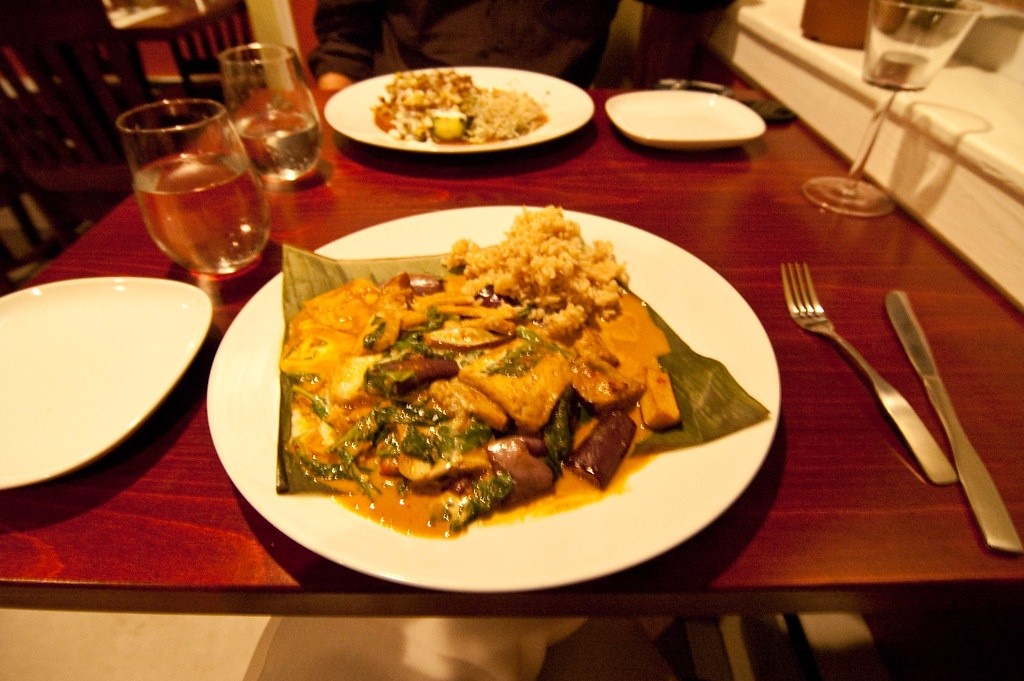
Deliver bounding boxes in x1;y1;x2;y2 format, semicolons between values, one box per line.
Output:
116;0;244;81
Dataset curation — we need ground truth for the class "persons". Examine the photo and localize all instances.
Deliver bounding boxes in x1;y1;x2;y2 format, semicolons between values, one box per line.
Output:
307;0;736;91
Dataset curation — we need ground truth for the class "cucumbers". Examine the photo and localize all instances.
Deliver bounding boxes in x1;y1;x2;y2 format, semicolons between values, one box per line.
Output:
433;117;465;140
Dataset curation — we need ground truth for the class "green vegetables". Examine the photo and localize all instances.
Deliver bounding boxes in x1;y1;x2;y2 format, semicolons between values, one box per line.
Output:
288;306;590;531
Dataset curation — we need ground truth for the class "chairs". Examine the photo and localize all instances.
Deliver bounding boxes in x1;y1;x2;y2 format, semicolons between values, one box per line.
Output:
0;1;162;194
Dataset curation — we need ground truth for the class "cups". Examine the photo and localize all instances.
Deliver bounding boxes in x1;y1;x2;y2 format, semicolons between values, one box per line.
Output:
216;40;321;183
115;98;271;275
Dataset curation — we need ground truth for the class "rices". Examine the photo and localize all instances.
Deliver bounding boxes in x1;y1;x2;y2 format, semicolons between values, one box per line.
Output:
440;203;630;336
463;88;545;142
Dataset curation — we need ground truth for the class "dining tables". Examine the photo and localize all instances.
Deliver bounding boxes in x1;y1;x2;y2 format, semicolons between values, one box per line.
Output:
0;82;1024;619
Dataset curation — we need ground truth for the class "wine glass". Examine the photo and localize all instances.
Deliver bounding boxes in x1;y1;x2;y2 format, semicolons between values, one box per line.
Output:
804;0;986;218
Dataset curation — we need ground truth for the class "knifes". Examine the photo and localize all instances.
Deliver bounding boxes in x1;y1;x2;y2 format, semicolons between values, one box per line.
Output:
882;289;1024;555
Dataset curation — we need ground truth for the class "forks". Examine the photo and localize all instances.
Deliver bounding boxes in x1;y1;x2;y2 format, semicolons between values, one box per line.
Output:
780;260;959;487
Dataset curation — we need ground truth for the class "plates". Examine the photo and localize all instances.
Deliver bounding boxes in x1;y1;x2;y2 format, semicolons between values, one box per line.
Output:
0;277;215;493
204;202;790;595
321;64;596;152
606;91;765;149
